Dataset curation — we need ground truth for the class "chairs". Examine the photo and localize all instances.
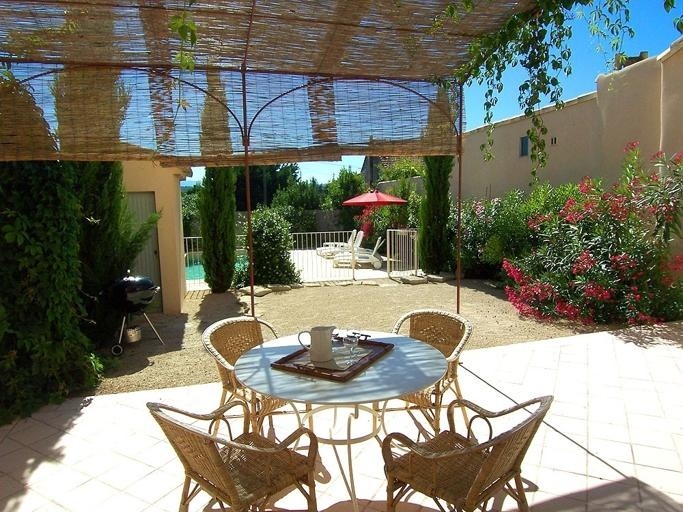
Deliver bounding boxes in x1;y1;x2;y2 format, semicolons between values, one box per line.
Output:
315;229;386;271
201;308;478;512
382;395;554;512
147;399;318;512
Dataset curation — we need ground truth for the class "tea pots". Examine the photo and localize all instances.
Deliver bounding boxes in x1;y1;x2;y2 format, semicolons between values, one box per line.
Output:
298;326;337;363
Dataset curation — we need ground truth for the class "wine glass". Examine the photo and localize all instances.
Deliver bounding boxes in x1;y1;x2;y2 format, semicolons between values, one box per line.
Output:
347;327;362;355
345;337;359;365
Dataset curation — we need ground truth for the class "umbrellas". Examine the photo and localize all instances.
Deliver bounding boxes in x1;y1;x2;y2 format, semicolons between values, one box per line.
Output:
342;188;408;237
